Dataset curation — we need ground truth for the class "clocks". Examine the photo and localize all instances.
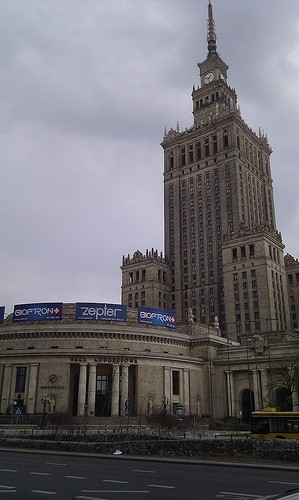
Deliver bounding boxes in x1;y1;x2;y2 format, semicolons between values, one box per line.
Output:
203;71;215;84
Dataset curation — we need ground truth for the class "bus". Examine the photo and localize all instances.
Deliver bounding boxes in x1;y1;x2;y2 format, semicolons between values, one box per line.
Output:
251;407;299;440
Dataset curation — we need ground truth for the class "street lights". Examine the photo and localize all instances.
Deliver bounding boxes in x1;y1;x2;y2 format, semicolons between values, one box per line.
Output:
41;395;49;431
